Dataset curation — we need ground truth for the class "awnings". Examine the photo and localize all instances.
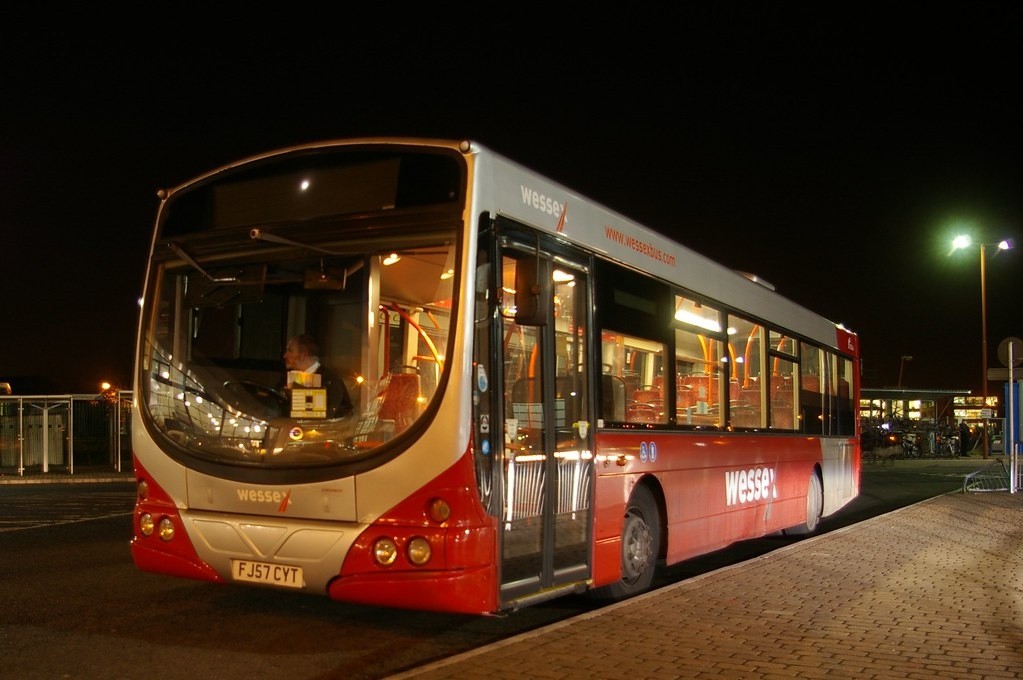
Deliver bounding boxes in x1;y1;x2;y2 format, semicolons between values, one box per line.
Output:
859;388;972;422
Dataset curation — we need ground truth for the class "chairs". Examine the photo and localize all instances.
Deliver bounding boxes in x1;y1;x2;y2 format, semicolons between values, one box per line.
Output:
624;370;849;430
381;364;421;419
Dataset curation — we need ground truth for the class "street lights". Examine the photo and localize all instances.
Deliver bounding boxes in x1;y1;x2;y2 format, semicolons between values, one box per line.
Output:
953;235;1012;461
897;355;914;390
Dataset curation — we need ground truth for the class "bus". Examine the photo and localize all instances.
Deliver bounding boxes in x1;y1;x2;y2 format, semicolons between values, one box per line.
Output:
130;137;865;622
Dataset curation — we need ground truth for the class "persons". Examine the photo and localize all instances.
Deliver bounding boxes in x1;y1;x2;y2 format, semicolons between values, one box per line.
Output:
283;334;354;420
959;419;974;456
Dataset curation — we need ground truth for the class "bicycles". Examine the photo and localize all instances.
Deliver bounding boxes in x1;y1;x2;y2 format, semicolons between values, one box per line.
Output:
861;409;960;459
